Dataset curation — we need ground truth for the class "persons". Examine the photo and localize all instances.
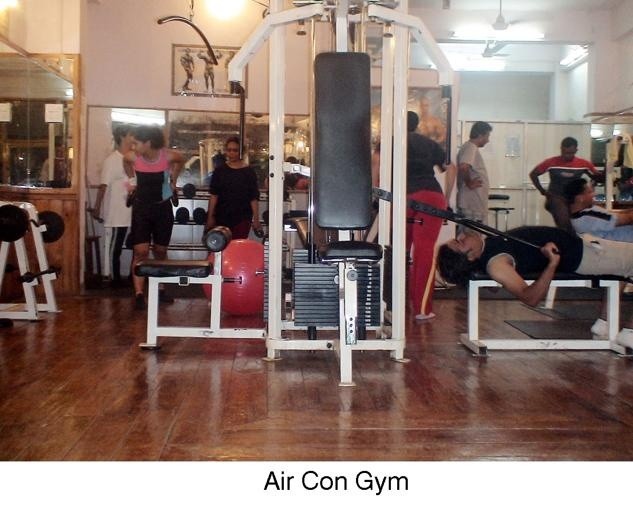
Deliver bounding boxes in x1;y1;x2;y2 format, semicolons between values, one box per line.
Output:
371;110;457;322
528;136;602;233
263;155;310;215
615;177;633;202
435;224;632;350
38;136;73;188
89;125;137;290
404;321;438;399
124;124;184;310
561;176;633;244
454;121;492;239
202;153;225;184
200;136;261;256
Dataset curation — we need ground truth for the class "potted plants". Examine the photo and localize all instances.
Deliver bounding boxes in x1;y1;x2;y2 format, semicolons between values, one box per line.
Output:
170;43;250;101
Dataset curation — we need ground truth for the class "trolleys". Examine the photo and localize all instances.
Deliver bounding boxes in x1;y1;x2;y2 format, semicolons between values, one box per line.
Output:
474;42;511;59
492;0;523;31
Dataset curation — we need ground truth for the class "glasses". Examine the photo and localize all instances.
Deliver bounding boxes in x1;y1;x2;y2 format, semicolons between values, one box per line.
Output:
558;45;590;72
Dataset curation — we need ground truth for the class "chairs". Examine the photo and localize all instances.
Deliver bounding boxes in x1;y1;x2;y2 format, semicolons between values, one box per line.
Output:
135;288;173;310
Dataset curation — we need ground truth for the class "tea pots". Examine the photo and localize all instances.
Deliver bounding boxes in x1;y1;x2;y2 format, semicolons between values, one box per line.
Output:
0;53;80;194
166;108;310;194
589;122;633;209
83;104;167;188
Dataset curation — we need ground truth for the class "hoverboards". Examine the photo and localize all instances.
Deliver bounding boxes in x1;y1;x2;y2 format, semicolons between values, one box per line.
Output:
0;203;65;244
19;263;61;283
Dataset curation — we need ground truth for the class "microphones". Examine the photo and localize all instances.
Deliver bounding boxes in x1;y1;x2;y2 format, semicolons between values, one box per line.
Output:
316;241;385;388
128;258;213;348
